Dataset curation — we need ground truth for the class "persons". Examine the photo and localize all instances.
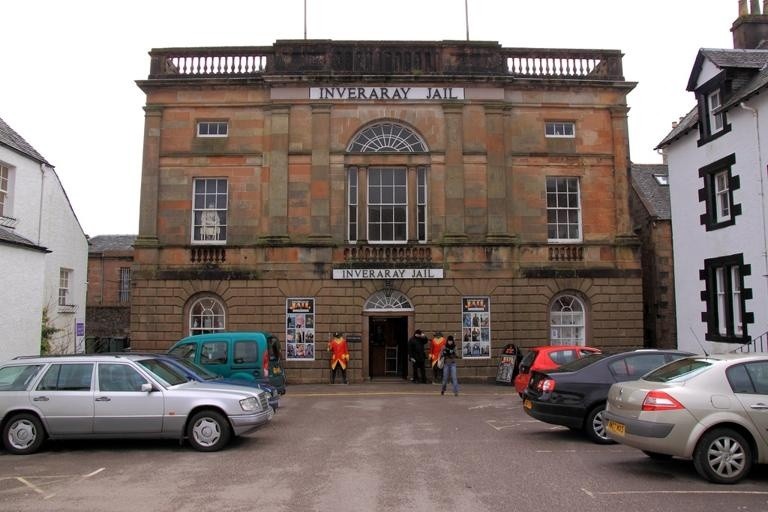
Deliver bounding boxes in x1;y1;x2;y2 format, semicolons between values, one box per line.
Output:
439;334;461;397
471;313;479;327
287;315;291;328
464;314;470;326
430;330;445;383
325;331;349;384
472;328;476;341
406;329;429;384
476;329;479;336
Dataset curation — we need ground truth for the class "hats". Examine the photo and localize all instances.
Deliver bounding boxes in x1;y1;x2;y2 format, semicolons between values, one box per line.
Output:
434;331;443;337
333;331;342;337
414;329;421;336
447;336;454;345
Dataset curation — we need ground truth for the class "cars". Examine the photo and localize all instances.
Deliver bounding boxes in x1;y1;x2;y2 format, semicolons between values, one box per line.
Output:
160;352;278;418
519;347;714;446
599;348;768;482
509;343;639;401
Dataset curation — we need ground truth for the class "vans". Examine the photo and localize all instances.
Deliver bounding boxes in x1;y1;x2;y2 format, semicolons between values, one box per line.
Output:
0;354;277;456
162;328;288;399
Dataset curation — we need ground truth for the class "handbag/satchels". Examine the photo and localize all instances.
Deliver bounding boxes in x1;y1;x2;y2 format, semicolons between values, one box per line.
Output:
437;357;445;369
